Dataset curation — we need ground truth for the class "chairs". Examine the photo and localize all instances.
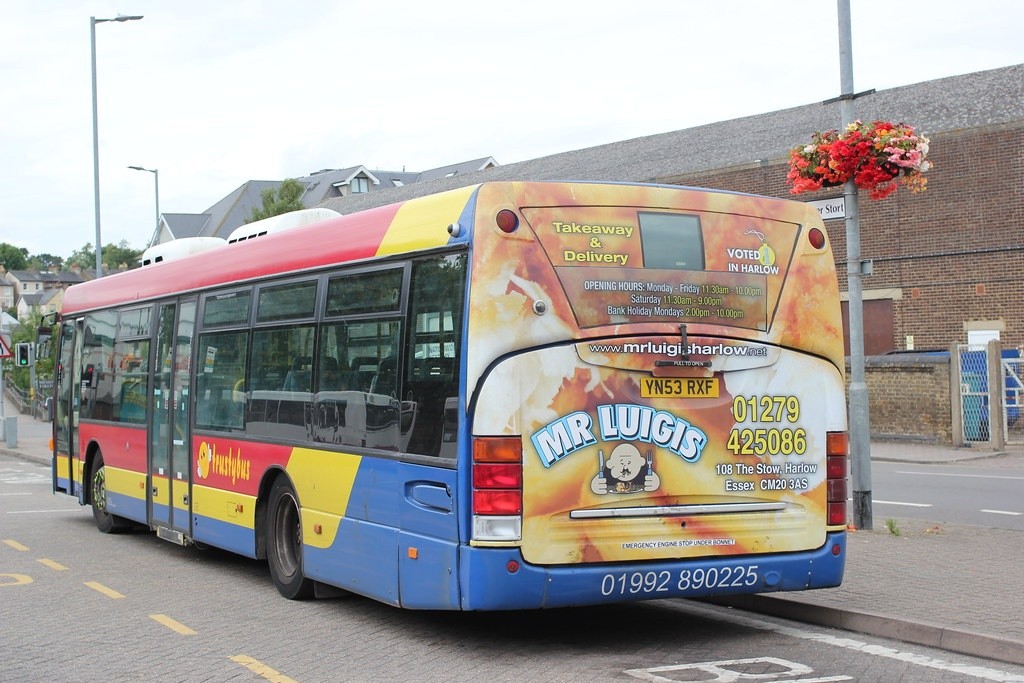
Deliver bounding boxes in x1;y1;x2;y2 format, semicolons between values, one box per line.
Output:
233;355;455;426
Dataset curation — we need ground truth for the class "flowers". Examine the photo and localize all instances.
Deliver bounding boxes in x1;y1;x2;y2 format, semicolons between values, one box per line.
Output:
785;119;935;201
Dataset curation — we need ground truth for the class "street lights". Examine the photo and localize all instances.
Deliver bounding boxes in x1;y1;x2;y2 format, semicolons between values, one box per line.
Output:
89;14;144;280
127;165;160;245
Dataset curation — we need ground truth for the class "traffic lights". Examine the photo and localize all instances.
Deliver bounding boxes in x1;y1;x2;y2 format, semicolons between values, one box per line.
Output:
15;343;29;367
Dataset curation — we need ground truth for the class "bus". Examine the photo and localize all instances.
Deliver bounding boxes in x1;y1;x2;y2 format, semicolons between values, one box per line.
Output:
35;179;852;613
